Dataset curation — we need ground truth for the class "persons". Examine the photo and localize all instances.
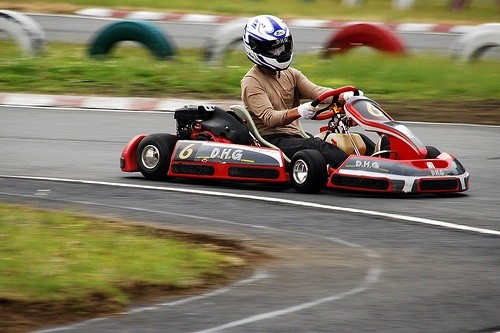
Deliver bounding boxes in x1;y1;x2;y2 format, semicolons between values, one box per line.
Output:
240;15;390;170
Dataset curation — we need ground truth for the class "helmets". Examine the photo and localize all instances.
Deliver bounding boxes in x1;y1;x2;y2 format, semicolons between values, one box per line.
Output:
243;15;293;71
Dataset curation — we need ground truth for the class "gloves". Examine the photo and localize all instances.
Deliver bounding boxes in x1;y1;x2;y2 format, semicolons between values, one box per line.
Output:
343;89;364;101
298;102;319;120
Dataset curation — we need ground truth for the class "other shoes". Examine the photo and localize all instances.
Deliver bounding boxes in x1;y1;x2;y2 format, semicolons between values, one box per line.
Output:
373;134;391;159
